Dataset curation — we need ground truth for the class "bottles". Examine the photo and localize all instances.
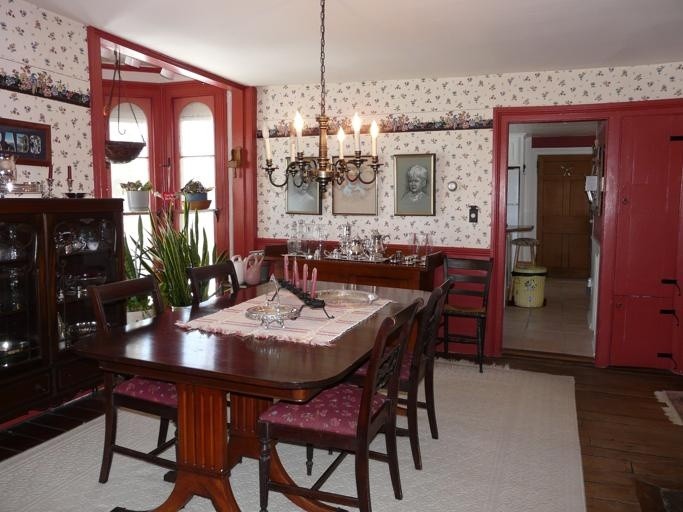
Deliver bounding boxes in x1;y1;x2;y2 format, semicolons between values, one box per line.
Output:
285;219;305;255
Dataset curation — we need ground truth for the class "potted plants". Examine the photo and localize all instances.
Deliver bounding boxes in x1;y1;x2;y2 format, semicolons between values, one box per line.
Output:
120;179;153;213
120;214;155;322
132;196;226;312
178;180;213;202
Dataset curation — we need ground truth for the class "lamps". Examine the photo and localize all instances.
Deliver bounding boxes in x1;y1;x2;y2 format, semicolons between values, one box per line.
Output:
257;1;383;194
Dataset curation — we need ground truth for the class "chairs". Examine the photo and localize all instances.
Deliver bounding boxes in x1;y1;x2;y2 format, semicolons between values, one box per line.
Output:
352;276;451;473
253;296;427;512
80;272;238;494
428;253;497;376
183;259;244;306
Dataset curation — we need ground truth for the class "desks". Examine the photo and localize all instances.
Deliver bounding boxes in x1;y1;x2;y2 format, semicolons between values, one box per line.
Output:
67;277;439;512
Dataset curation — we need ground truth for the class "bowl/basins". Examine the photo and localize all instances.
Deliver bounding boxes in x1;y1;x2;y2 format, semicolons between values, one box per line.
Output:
0;339;30;357
63;192;89;199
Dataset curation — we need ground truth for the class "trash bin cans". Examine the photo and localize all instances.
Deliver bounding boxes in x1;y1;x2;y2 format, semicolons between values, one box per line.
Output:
511;266;547;308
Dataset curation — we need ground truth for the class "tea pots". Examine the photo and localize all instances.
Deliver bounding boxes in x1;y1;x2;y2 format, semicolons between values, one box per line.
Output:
228;253;266;285
361;229;391;262
342;234;365;258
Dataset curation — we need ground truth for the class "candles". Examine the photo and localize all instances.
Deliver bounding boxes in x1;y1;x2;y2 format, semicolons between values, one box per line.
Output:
66;164;72;179
278;252;320;301
47;163;52;180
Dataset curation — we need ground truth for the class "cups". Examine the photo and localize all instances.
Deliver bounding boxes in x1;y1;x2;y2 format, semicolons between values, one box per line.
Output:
390;250;430;266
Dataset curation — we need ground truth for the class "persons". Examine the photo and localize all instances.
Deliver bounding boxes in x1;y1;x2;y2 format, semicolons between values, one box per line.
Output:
399;164;428;202
289;178;313;202
340;165;370;197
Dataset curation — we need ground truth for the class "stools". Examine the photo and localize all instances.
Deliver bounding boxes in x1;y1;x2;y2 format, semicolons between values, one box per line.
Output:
508;236;542;301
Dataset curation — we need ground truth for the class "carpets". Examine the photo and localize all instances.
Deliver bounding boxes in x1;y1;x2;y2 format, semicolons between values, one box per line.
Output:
654;387;682;426
0;354;592;512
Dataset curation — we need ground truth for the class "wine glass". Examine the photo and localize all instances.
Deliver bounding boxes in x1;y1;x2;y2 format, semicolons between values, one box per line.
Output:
303;223;327;258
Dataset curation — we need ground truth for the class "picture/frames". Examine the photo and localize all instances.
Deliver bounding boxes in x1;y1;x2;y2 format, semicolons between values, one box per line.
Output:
0;116;57;167
391;151;440;218
329;154;380;215
282;157;324;216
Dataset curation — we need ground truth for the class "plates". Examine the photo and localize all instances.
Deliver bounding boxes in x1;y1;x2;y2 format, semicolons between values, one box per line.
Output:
73;319;103;334
309;289;378;306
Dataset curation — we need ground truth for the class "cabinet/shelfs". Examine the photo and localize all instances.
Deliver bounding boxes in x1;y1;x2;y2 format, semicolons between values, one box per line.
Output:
50;195;131;412
259;240;445;290
1;195;57;430
